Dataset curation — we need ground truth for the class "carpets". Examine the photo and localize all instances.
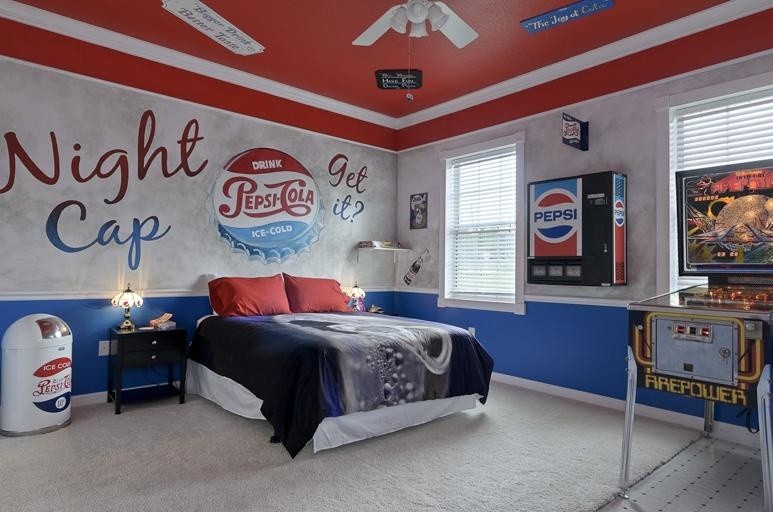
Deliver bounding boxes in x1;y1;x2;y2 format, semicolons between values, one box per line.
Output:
0;380;704;512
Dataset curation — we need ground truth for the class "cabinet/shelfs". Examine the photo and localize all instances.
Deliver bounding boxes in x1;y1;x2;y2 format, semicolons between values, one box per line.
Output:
526;171;627;286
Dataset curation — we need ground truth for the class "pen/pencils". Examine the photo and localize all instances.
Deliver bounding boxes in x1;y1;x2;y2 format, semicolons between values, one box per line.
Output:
137;328;155;330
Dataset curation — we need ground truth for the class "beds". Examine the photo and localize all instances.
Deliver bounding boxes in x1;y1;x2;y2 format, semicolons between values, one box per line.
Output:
184;312;494;459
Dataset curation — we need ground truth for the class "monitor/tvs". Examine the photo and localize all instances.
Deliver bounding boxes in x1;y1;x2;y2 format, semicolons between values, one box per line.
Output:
676;159;773;276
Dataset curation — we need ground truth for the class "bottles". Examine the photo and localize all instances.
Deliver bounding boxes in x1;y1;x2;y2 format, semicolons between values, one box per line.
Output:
403;248;430;287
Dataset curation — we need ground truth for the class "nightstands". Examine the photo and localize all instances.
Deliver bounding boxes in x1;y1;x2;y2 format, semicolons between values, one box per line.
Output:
107;325;187;414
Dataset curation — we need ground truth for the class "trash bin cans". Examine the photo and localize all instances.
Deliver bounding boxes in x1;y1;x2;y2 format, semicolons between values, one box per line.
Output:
1;313;72;437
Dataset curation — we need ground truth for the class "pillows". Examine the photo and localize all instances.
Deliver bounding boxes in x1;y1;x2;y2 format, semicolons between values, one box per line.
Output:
207;270;353;319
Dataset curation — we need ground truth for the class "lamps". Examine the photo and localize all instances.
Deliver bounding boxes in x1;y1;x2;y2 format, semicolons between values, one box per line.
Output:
389;0;449;38
111;283;144;329
346;281;366;312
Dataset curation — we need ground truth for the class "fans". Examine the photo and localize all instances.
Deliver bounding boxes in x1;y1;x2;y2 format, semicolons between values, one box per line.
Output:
352;1;479;49
